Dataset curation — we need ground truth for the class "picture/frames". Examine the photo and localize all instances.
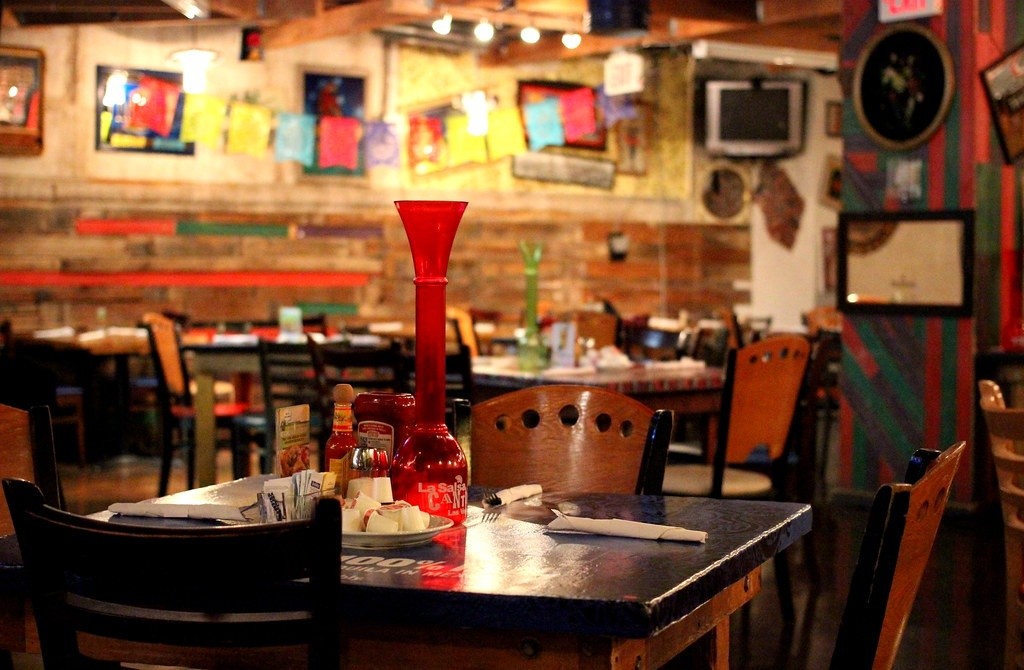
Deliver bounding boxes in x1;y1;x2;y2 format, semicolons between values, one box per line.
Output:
516;77;606;150
0;45;46;156
294;63;373;186
836;211;974;319
94;63;196;157
854;22;954;151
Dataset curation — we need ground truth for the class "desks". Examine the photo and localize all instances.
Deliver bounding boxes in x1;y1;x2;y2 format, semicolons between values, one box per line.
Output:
466;355;724;471
0;472;811;670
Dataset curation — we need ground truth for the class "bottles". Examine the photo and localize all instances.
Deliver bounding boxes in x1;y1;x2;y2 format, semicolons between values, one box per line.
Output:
518;241;547;367
389;200;468;524
325;384;362;500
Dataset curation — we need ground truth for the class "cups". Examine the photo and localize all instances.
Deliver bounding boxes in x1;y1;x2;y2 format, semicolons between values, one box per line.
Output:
354;393;415;459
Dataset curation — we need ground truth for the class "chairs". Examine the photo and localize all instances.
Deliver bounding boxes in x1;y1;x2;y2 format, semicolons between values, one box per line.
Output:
0;320;1024;670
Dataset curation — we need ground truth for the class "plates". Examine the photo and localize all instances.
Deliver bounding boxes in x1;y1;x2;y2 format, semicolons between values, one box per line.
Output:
342;516;454;550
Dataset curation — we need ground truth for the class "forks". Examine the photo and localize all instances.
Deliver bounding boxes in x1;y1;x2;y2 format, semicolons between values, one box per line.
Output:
239;503;258;513
482;489;501;505
551;509;565;518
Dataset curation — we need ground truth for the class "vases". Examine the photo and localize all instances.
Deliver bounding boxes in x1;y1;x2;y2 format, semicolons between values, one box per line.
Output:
519;242;548;370
390;201;469;492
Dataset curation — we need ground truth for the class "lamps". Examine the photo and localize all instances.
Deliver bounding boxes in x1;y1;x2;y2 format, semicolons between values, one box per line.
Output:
432;11;581;48
